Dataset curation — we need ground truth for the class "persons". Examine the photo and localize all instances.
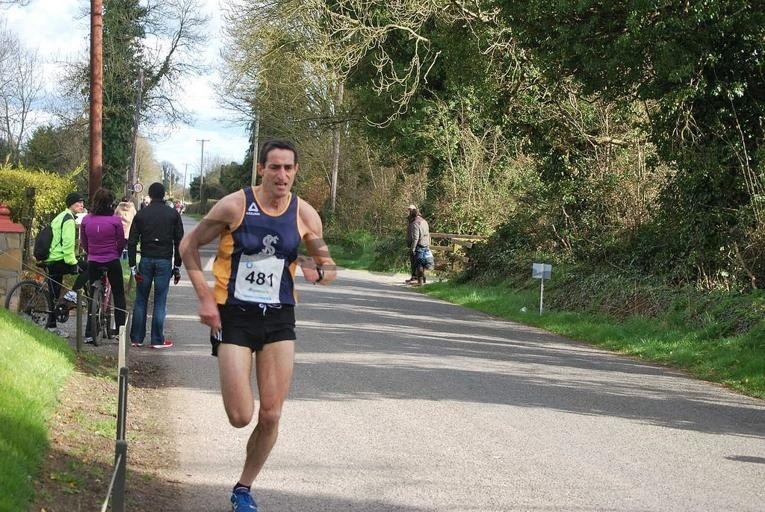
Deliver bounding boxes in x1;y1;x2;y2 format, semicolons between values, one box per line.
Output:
178;141;337;512
72;189;126;344
33;192;86;338
128;183;183;349
113;197;186;259
405;205;434;286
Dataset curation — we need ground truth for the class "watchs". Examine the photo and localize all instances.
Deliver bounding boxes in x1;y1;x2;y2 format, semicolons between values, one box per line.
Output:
316;267;324;282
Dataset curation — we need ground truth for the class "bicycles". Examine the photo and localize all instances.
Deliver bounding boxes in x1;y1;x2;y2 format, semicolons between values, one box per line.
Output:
5;252;120;346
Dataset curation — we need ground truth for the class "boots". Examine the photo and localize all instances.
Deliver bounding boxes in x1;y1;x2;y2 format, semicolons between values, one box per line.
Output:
405;276;427;287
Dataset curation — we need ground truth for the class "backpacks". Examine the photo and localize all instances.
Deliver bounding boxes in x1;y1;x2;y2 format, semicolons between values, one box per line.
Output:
32;224;54;261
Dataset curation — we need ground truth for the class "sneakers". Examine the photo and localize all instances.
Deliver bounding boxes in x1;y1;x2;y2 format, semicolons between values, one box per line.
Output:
63;289;87;307
228;487;260;512
151;339;173;348
47;327;70;338
131;340;143;347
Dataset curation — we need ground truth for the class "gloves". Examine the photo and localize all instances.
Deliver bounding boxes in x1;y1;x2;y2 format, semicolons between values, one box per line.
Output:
170;265;182;285
130;265;139;277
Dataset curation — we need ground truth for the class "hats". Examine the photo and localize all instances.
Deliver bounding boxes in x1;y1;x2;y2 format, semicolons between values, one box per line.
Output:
148;181;166;199
65;191;85;209
407;204;416;209
